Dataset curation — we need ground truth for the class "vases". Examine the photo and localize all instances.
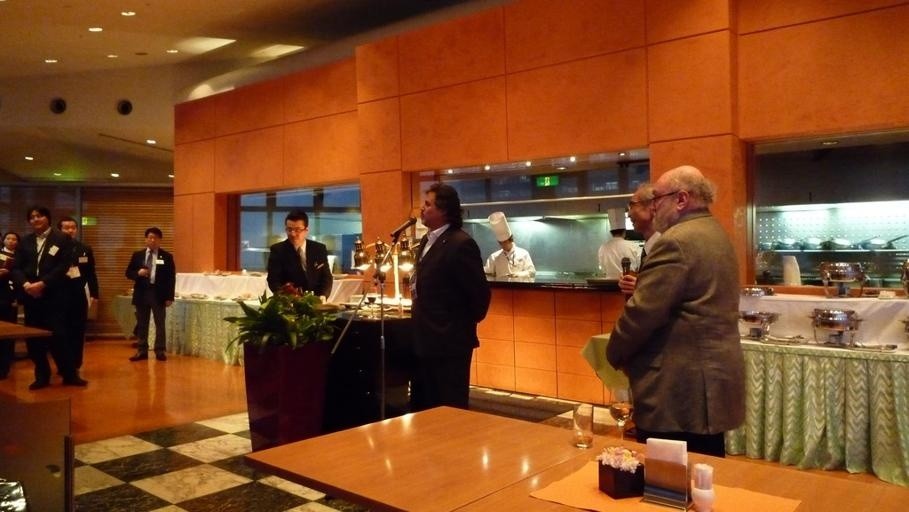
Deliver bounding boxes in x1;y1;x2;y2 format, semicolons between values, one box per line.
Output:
243;342;327;453
598;462;644;499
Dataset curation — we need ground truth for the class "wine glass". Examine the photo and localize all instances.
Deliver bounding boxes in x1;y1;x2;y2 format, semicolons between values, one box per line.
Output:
610;401;633;451
367;296;377;319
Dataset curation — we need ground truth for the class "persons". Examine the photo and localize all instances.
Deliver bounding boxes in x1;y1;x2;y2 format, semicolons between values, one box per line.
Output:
12;205;90;389
624;181;662;264
606;165;748;458
1;231;20;378
482;211;537;278
125;225;176;361
266;208;333;304
597;207;642;279
50;216;100;375
407;181;492;412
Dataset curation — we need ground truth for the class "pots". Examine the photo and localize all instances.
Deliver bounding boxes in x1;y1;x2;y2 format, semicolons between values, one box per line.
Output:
772;234;909;249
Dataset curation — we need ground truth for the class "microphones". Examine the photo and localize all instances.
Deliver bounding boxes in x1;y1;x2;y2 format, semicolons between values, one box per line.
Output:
390;216;417;236
620;256;632;275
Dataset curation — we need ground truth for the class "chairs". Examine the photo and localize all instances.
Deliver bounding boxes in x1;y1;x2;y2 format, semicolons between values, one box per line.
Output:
1;398;75;512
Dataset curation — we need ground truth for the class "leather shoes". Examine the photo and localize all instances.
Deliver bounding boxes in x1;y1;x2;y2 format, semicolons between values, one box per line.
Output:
29;381;48;390
129;351;148;360
64;379;87;385
156;352;167;361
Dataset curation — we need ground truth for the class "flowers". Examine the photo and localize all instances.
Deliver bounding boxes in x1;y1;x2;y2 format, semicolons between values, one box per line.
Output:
221;282;339;353
595;446;639;474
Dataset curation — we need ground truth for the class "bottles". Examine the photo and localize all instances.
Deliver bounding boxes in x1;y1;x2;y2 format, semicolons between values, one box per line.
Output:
691;464;714;512
399;298;403;312
403;274;410;297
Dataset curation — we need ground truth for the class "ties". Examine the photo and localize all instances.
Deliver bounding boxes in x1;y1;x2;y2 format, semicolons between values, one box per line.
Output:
145;250;153;285
298;247;308;272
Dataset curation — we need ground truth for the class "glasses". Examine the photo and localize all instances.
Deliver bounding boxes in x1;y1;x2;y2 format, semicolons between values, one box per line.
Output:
651;192;679;202
627;199;646;209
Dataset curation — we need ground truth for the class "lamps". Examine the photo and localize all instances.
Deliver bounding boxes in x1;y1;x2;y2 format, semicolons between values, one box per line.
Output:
351;237;415;272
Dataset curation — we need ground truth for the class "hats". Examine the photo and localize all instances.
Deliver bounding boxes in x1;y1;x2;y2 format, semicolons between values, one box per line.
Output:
607;208;626;231
488;212;511;242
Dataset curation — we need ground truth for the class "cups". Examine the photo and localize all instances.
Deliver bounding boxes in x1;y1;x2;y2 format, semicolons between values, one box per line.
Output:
573;402;594;447
